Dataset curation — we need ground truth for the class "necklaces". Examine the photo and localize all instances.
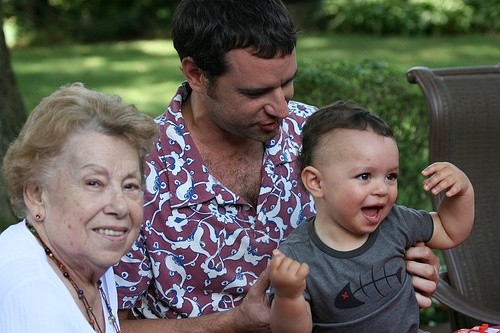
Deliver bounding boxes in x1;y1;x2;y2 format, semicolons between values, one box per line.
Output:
25;219;120;333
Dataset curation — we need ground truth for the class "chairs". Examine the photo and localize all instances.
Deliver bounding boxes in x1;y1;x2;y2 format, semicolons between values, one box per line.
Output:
407;65;500;331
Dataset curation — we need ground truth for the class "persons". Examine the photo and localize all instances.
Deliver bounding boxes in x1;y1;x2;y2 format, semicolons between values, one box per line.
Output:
112;1;440;333
0;83;159;333
267;99;475;333
